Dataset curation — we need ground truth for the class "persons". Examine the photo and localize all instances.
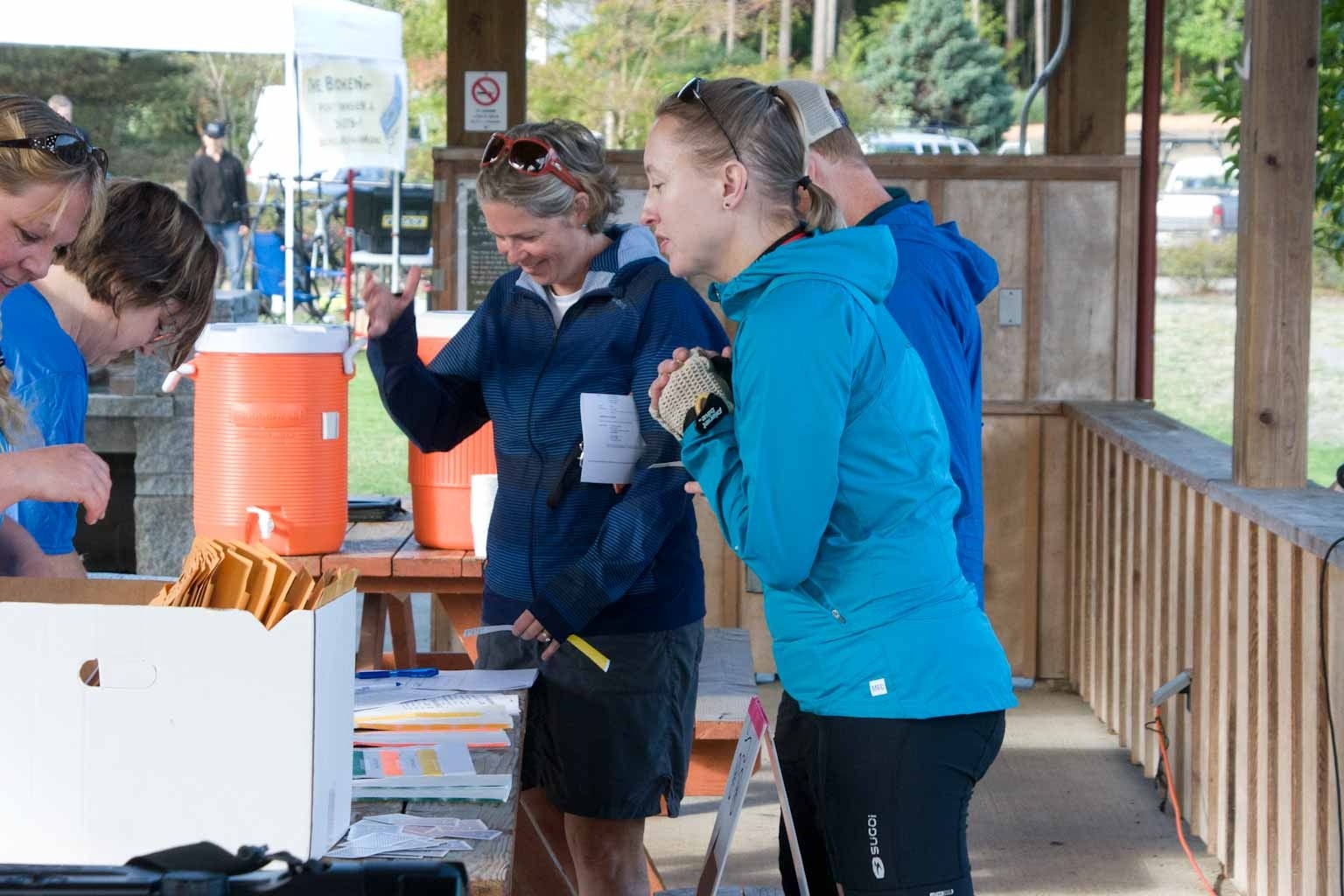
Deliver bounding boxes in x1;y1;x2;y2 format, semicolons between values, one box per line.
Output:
187;121;251;291
1;89;111;576
771;81;1004;610
362;117;735;896
641;76;1017;896
48;95;91;146
1;178;219;576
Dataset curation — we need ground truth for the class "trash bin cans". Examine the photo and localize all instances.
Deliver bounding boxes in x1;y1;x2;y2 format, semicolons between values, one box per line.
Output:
254;231;309;301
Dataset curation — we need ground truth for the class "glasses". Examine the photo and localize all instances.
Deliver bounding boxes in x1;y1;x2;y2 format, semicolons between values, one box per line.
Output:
677;75;749;190
153;300;180;351
480;131;584;194
0;132;110;179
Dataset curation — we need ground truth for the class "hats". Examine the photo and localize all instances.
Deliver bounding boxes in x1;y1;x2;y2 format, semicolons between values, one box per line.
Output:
770;80;848;146
205;121;226;139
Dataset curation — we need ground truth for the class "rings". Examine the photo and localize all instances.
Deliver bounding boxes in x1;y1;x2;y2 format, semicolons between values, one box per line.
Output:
542;632;551;642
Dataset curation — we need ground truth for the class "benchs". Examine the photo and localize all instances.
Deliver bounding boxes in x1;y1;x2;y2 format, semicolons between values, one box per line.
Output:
673;628;763;797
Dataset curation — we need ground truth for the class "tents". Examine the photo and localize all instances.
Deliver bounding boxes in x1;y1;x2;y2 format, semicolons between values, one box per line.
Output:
0;0;408;323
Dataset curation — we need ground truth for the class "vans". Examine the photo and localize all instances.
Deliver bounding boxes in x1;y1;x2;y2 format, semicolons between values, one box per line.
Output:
855;128;982;156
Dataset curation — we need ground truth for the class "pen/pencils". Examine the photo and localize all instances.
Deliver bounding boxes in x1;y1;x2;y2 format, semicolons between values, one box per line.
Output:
354;667;440;679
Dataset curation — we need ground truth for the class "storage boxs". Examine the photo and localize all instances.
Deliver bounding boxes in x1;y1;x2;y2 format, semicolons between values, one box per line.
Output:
0;574;360;866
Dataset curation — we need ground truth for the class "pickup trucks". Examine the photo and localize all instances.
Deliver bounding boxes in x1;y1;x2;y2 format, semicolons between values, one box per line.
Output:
1154;155;1241;233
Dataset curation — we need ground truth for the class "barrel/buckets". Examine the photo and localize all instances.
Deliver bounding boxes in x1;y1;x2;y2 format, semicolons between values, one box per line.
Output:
160;322;369;558
407;310;496;550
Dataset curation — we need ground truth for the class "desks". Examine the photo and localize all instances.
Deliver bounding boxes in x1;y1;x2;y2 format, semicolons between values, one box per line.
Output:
323;671;528;895
349;248;436;294
277;501;666;895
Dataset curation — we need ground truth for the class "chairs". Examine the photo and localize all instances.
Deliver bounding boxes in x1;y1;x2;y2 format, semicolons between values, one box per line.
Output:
248;228;345;325
